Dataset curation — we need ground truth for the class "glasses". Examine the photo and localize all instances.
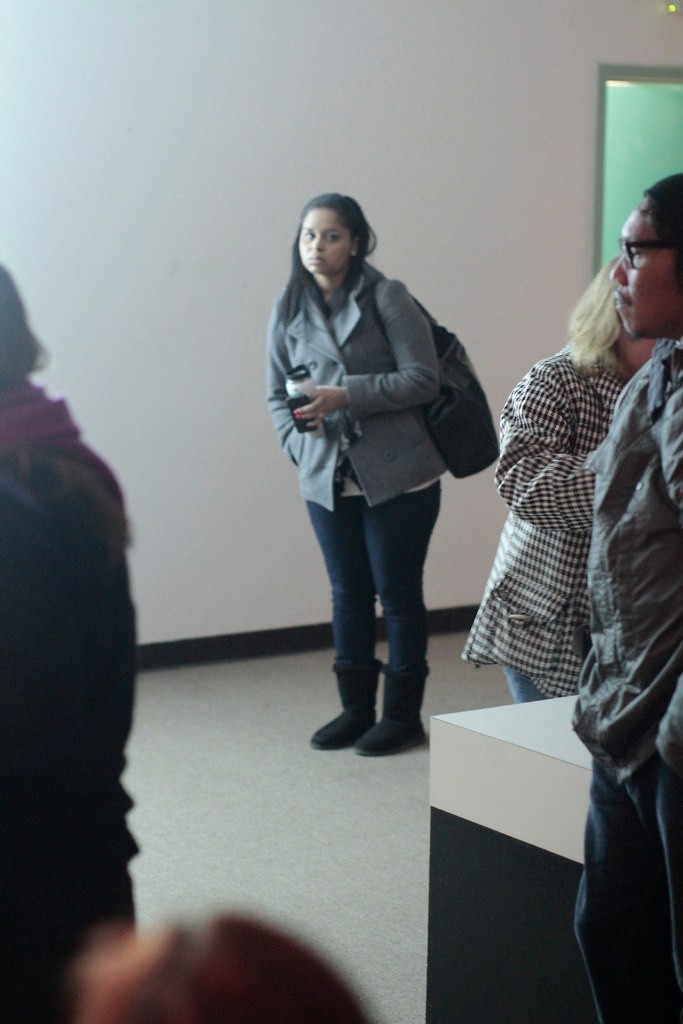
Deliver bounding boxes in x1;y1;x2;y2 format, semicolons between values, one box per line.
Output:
620;238;669;267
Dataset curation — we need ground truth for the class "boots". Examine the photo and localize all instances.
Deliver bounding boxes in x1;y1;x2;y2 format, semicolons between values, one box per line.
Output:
311;659;428;757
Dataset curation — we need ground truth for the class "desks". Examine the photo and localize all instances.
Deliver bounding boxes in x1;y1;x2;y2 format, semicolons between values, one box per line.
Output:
422;695;599;1024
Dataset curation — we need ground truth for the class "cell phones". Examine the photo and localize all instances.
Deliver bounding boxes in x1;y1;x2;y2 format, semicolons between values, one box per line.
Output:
286;394;318;433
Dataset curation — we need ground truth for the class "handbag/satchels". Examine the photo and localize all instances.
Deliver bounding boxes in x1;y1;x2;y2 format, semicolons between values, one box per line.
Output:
370;277;499;478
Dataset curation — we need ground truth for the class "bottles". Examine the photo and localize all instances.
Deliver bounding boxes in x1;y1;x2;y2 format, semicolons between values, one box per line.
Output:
285;364;325;439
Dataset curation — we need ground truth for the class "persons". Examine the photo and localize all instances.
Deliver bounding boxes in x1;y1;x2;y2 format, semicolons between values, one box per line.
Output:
463;257;656;706
71;911;366;1024
571;174;683;1024
0;262;142;1024
266;193;448;755
